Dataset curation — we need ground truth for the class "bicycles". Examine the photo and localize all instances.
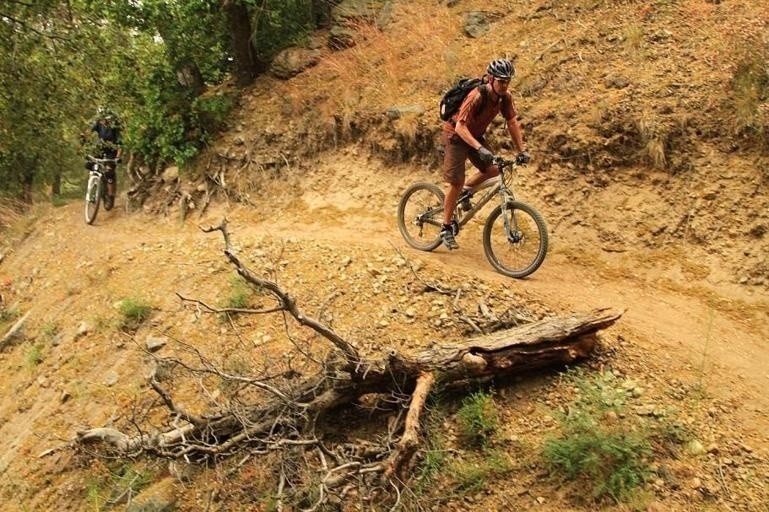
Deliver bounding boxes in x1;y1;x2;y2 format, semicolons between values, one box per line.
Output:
396;154;547;278
77;151;119;225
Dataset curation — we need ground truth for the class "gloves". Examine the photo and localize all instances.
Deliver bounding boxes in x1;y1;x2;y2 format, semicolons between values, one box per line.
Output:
477;146;493;161
115;157;121;164
516;152;531;163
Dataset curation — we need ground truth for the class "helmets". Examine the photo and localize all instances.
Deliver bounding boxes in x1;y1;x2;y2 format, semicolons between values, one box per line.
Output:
486;59;514;78
97;106;111;119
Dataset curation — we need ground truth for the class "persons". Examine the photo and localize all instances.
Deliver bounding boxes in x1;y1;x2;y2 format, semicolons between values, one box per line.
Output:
76;104;126;210
437;58;531;250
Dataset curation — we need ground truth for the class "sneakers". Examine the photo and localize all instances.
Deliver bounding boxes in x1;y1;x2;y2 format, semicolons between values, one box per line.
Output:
103;194;115;210
439;228;460;249
458;188;473;210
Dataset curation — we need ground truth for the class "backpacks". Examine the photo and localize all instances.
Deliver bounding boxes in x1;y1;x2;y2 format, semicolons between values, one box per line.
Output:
439;75;508;121
